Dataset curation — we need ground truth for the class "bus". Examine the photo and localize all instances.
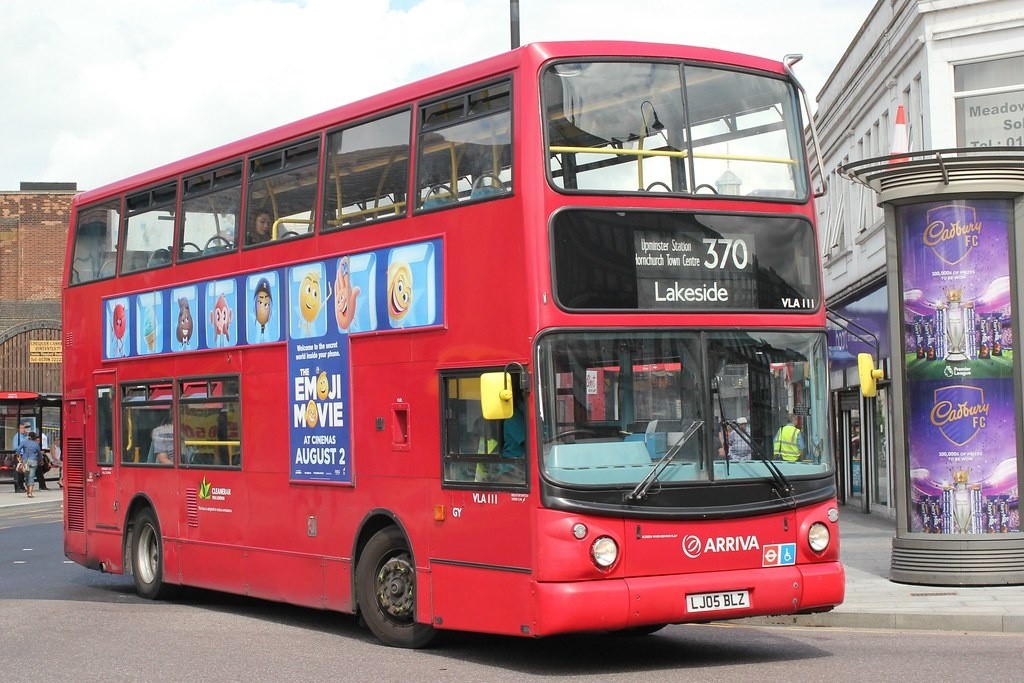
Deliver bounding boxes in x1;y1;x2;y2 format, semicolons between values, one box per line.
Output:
58;38;885;650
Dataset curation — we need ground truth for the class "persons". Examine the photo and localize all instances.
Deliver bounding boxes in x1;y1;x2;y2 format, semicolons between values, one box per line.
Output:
50;437;63;487
500;378;549;492
717;418;733;458
30;436;50;491
151;402;188;465
770;413;806;462
13;422;27;493
728;417;752;462
17;432;44;498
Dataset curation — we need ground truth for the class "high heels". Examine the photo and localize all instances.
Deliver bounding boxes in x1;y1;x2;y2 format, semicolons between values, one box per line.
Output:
39;483;49;490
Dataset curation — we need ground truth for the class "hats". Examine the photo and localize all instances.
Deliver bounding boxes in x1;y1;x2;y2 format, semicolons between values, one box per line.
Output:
737;417;748;425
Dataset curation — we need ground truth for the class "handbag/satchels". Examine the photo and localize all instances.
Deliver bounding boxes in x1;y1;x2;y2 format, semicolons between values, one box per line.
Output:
16;462;29;473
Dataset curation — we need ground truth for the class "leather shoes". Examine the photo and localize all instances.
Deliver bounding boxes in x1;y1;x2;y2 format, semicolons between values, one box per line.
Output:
15;488;26;493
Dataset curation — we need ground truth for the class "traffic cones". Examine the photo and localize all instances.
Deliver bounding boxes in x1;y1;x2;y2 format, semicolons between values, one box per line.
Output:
886;106;911;171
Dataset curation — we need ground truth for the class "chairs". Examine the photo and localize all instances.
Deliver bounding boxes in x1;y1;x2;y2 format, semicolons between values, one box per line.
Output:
98;174;507;279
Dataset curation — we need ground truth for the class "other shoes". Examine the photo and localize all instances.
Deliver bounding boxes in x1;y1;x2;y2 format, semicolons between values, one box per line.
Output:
57;481;63;488
28;494;34;498
